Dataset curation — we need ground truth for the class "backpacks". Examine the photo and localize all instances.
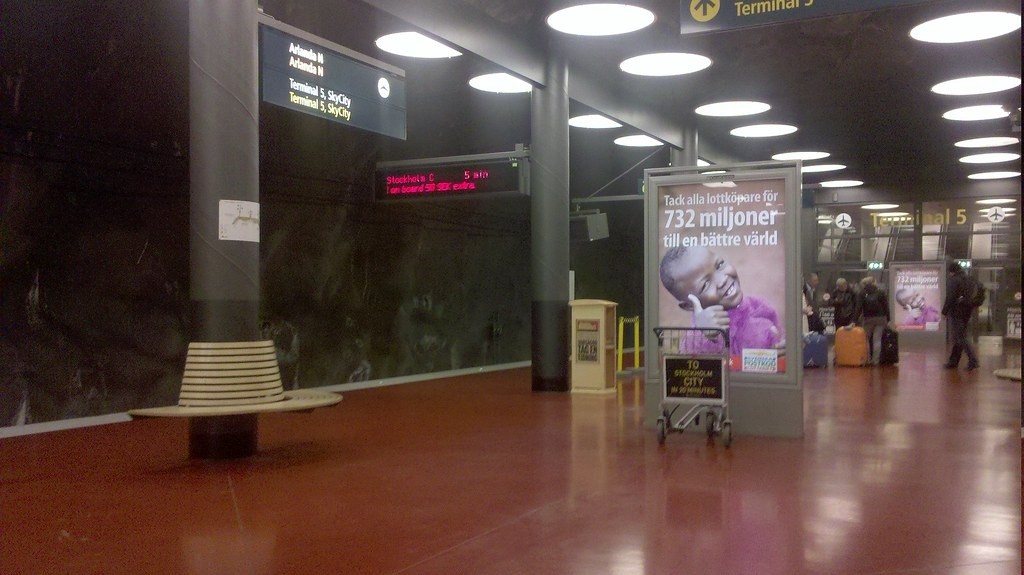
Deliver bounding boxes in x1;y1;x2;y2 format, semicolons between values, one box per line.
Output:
955;273;985;309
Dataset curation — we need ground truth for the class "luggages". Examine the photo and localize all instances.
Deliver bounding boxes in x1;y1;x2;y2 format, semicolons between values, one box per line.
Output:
835;323;870;367
802;331;828;368
881;327;900;364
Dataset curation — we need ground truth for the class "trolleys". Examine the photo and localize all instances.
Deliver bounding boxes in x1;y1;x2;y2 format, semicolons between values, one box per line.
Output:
653;326;733;447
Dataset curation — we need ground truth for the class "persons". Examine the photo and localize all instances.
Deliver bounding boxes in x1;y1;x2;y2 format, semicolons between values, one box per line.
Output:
942;262;980;369
896;289;940;325
802;272;890;366
660;245;786;358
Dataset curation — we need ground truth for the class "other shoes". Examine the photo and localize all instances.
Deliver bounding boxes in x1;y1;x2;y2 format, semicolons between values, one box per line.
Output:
943;363;959;369
966;362;979;372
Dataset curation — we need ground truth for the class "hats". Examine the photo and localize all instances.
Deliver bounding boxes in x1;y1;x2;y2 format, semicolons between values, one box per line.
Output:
949;262;961;272
